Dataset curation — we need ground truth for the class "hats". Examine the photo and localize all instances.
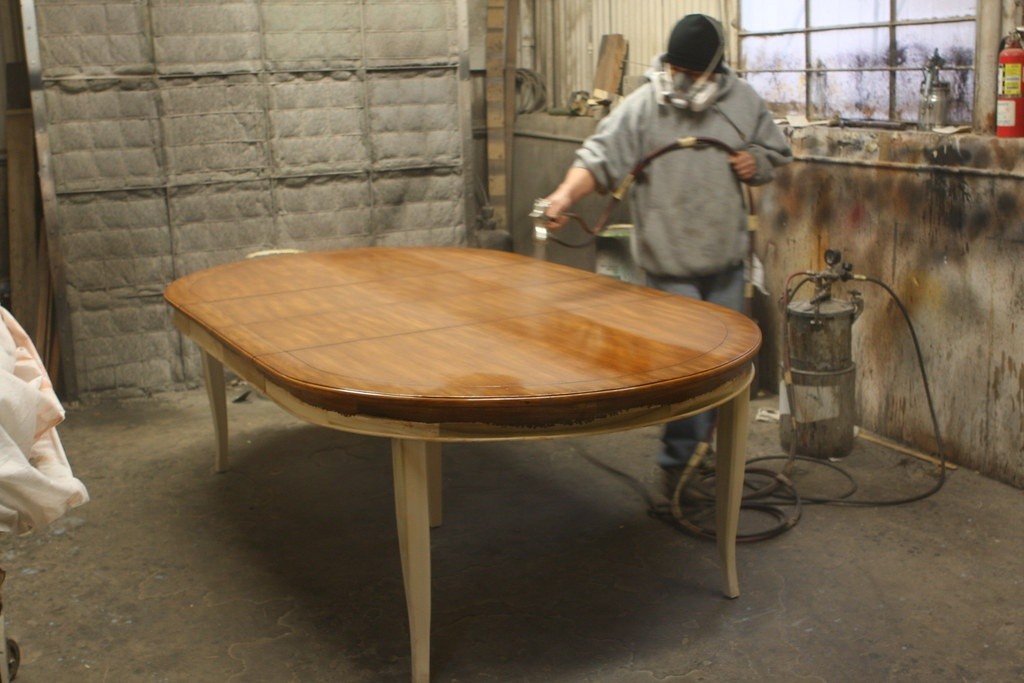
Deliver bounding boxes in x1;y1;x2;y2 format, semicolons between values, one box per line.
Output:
659;14;731;76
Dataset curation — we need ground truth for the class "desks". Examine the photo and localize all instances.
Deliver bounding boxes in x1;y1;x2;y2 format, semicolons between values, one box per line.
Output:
161;246;764;683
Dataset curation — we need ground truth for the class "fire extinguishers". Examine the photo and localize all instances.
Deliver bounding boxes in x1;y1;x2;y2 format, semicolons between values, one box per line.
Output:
994;27;1024;139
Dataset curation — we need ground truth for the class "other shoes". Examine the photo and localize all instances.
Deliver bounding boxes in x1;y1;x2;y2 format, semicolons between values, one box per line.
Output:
661;444;719;503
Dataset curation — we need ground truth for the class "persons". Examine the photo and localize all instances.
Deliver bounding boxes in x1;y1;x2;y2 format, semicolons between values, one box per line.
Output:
530;13;794;510
